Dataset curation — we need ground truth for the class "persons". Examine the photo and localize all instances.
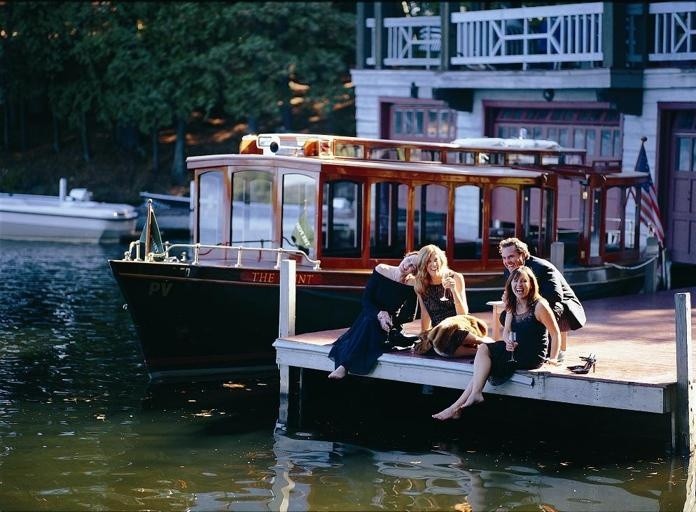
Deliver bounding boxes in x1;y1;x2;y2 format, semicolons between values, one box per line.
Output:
326;250;422;378
414;244;496;359
497;238;585;363
432;266;561;420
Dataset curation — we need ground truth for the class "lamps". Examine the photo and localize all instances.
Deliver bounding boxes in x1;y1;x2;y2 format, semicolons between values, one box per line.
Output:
410;82;418;98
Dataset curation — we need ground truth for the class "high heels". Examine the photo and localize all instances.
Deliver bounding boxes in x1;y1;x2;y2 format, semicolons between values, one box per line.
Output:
567;355;596;374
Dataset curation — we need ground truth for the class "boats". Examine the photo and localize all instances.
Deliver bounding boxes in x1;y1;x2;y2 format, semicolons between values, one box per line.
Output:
125;187;360;244
103;119;671;389
0;171;144;243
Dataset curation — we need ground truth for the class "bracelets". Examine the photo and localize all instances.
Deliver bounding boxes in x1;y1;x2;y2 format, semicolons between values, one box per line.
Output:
454;299;462;305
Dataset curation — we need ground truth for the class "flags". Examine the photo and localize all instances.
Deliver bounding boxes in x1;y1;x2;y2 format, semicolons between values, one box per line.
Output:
630;142;666;249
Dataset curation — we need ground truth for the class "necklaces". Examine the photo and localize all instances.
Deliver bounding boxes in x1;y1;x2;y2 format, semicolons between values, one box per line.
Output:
518;303;524;314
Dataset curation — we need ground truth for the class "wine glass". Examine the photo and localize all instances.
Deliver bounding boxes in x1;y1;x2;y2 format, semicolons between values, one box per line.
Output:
507;331;518;363
384;315;392;344
439;271;452;301
